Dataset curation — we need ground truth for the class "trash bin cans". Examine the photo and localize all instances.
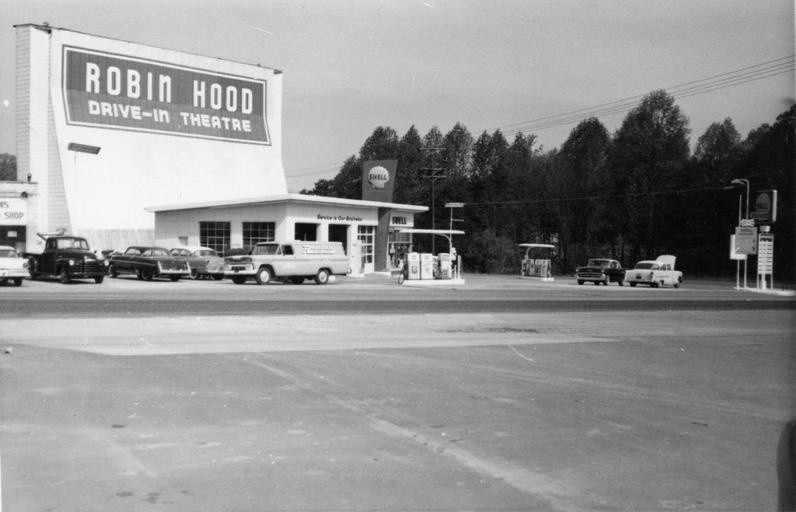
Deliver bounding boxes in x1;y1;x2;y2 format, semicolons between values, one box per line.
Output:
405;252;433;280
438;253;452;279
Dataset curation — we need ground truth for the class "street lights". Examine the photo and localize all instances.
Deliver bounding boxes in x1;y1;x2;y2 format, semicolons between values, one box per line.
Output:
731;177;749;289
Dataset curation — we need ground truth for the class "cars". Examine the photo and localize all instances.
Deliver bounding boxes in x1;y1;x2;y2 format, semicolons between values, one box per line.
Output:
0;245;29;286
109;245;226;281
574;254;683;288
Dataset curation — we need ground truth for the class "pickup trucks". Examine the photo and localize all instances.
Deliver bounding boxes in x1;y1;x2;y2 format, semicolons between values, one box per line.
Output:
18;236;108;283
226;240;349;286
